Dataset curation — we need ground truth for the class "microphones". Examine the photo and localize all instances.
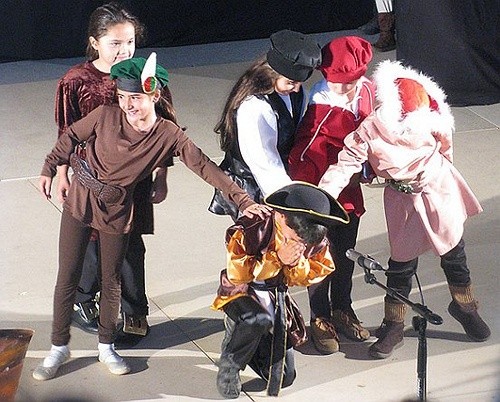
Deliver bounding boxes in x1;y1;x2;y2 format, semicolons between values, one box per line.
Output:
345;248;382;270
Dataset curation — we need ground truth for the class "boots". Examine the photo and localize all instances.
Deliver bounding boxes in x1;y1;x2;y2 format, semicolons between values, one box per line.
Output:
371;12;395;52
360;14;379;35
368;297;407;359
447;277;492;342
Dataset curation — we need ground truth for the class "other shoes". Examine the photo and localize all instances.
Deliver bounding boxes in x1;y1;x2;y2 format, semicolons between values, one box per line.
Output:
98;352;131;375
33;348;70;381
216;349;241;399
220;312;236;354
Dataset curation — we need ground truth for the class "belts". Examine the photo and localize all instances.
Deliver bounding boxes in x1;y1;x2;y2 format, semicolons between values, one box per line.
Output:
69;152;129;206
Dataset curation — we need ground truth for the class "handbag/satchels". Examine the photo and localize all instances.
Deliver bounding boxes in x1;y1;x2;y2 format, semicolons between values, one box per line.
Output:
207;157;261;223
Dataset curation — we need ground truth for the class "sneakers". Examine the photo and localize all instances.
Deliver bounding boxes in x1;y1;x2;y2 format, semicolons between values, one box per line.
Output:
123;310;148;337
332;306;370;342
70;299;99;335
307;315;341;355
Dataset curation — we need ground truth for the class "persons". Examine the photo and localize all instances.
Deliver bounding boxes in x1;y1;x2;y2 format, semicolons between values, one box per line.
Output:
33;52;273;381
369;0;397;53
287;36;376;355
55;2;177;343
319;59;491;360
212;180;351;399
208;28;321;225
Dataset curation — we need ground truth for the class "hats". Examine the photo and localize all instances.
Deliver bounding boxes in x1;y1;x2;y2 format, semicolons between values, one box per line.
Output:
373;58;455;137
266;29;323;83
316;36;373;84
263;180;351;225
110;51;169;94
